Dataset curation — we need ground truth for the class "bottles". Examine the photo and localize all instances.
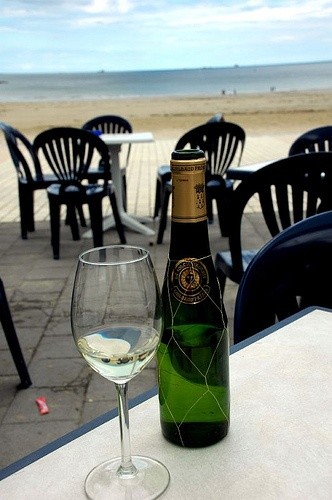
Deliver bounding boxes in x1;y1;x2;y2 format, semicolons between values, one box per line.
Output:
155;148;229;449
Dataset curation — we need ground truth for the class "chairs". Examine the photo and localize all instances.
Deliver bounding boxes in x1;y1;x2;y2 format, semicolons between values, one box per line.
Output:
32;124;128;266
153;114;332;345
0;121;77;241
65;114;133;227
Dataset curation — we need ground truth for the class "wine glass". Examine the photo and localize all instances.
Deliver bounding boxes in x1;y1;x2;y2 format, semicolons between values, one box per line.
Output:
70;245;170;500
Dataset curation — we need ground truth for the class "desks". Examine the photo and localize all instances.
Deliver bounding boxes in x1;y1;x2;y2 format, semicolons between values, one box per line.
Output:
79;131;156;239
0;305;332;500
